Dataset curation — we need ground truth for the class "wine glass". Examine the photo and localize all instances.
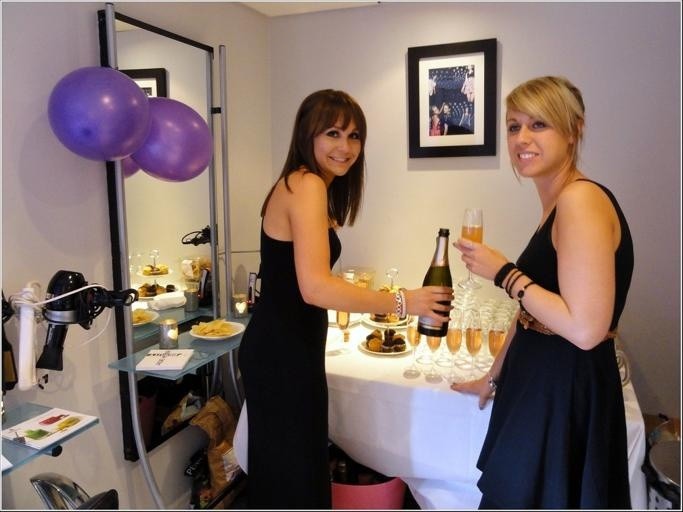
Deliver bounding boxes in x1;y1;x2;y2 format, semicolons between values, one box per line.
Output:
458;207;484;291
335;310;349;353
406;291;516;386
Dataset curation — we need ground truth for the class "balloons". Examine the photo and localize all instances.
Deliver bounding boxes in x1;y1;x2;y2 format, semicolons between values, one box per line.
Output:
120;157;140;178
128;96;214;184
44;63;152;166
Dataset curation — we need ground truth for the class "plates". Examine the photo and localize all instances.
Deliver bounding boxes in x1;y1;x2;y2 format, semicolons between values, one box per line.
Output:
189;322;246;342
132;310;159;328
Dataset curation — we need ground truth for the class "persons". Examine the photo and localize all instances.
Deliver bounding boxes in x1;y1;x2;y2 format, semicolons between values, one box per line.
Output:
232;85;458;511
444;71;635;512
428;101;474;137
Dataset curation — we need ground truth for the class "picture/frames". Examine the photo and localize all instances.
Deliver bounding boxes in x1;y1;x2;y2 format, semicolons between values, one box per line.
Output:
408;35;500;160
119;66;168;98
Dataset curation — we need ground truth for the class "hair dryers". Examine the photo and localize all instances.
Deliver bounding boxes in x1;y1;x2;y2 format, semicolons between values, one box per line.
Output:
181;224;212;246
36;270;139;370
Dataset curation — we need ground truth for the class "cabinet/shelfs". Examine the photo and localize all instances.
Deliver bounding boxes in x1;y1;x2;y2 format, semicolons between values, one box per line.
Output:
134;303;213;340
96;3;251;510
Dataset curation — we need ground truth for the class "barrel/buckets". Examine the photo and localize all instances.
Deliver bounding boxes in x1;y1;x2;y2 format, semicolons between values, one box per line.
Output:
331;476;405;511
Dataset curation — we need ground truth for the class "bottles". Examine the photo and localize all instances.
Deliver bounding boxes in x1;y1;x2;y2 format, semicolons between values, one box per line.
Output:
415;227;453;337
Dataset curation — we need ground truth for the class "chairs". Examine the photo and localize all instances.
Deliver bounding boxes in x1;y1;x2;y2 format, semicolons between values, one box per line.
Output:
28;473;119;512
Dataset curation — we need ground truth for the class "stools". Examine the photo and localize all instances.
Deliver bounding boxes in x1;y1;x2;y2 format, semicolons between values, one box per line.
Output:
641;439;682;509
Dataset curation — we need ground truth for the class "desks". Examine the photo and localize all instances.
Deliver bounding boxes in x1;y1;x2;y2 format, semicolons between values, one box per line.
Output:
1;402;99;475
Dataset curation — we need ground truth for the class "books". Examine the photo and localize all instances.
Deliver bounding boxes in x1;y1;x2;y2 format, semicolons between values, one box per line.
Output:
135;346;194;373
1;455;13;475
0;405;100;453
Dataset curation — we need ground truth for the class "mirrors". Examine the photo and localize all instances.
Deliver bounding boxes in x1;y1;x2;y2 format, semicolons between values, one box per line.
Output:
96;7;220;463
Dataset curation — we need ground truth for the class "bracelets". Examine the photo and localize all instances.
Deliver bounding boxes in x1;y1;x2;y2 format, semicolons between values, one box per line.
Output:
399;288;407;321
493;262;516;289
505;269;521;293
507;273;527;299
484;371;497;392
517;281;538;311
396;291;402;319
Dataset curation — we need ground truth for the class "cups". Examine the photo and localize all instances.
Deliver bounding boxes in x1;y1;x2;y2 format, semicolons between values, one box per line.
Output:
343;268;373;288
157;318;178;349
231;294;248;318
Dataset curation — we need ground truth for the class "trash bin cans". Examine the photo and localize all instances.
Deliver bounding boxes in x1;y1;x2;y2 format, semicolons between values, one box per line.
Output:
646;440;680;510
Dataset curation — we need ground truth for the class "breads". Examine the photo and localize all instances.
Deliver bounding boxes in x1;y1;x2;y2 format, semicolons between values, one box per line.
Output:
136;265;168;298
365;312;406;353
